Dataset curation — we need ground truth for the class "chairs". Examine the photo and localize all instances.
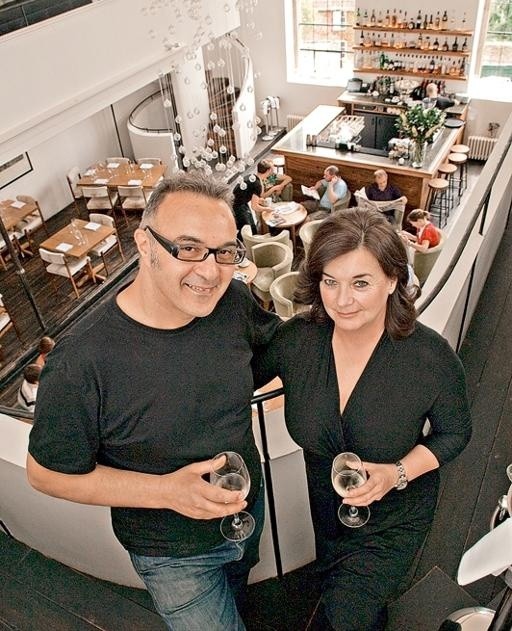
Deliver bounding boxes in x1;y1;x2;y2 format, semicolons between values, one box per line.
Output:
413;227;447;284
235;181;405;317
0;293;24;355
0;157;168;299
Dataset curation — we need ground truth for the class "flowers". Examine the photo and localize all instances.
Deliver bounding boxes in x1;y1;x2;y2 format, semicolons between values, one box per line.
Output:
393;105;444;163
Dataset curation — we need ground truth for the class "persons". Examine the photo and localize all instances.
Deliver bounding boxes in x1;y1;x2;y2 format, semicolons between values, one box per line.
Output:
233;159;293;237
398;209;439;253
18;336;55;412
26;174;285;631
365;169;400;224
295;165;348;237
423;83;460;112
251;206;472;631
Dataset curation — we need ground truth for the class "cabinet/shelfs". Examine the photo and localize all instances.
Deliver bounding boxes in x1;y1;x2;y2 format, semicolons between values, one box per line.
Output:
352;104;403;149
353;24;472;81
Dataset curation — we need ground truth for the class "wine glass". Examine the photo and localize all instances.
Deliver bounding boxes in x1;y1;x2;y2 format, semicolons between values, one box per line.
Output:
330;451;371;528
208;452;256;543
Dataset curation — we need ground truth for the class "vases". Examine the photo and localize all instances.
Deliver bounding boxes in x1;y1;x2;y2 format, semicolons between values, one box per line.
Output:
414;142;424;164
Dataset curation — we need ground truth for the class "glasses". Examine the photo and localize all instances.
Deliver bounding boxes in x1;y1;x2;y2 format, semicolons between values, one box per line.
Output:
142;225;246;265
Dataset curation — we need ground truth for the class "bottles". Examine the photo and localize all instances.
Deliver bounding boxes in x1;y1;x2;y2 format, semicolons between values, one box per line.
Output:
353;8;470;99
306;134;318;147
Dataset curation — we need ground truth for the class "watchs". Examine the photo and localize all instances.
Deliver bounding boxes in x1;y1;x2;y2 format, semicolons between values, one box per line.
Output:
394;460;409;490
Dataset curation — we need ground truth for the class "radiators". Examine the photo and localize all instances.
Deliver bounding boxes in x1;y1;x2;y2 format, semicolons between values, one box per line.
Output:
467;136;498;161
286;115;303;132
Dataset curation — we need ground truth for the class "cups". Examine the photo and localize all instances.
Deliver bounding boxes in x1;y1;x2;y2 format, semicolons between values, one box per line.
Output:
68;158;153;244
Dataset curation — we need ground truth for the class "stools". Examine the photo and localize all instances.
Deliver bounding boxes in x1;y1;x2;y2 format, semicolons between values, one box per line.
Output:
423;143;470;230
273;158;285;175
